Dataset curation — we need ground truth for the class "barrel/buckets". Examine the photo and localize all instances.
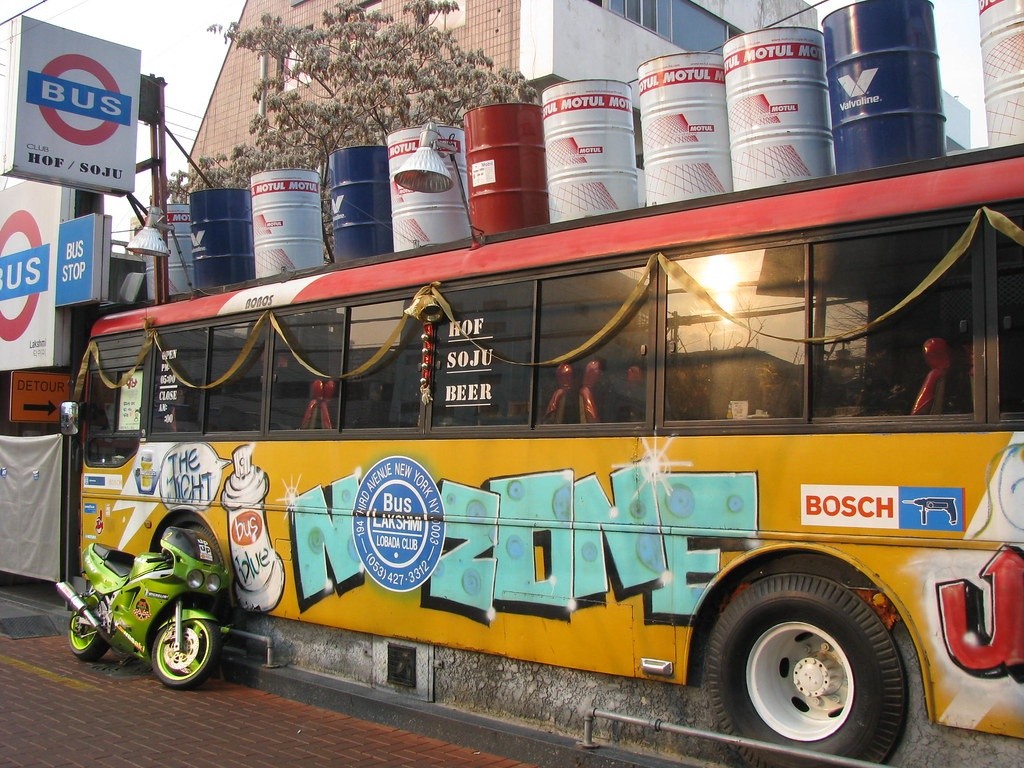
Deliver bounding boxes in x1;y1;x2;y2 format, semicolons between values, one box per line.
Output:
190;189;255;290
821;0;947;174
638;51;732;207
388;125;472;253
723;26;836;193
251;168;324;279
462;102;550;237
541;79;639;224
329;145;394;262
146;203;195;300
978;0;1024;148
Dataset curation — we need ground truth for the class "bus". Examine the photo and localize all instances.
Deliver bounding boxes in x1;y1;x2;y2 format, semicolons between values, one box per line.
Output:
74;143;1024;768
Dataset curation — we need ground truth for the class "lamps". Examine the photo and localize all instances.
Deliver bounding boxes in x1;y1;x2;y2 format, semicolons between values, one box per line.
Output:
393;122;485;252
125;207;202;301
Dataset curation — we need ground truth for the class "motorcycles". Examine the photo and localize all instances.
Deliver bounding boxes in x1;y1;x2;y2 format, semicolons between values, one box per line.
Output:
54;524;233;690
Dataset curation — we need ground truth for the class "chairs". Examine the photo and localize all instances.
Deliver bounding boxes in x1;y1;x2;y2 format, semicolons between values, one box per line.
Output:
289;337;963;431
